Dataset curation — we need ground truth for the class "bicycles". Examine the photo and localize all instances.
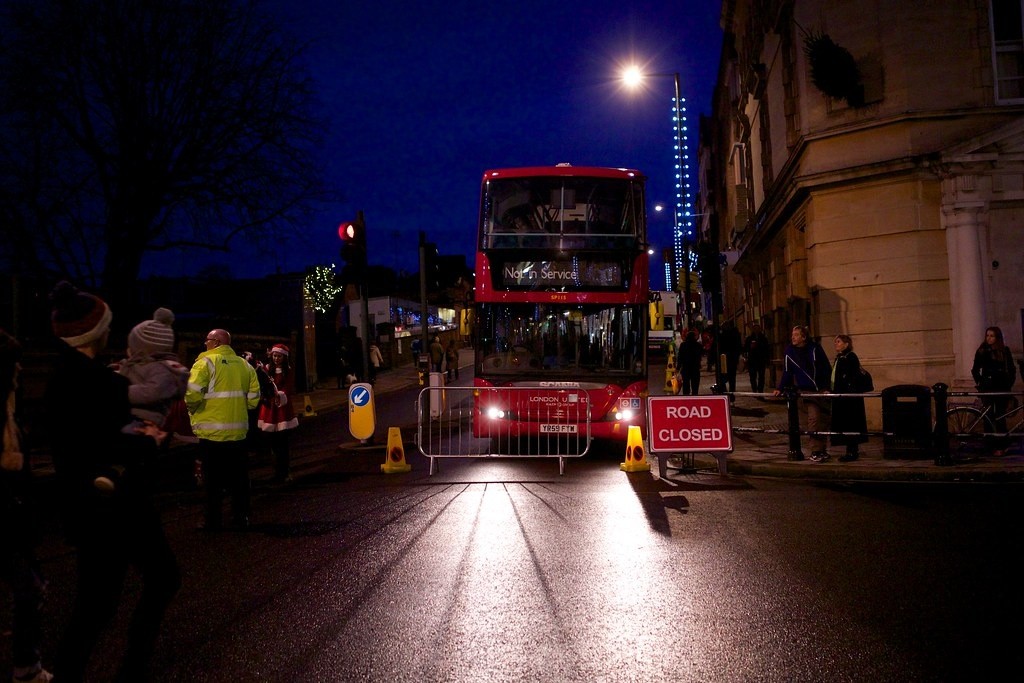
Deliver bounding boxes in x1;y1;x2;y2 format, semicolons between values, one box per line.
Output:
931;358;1024;465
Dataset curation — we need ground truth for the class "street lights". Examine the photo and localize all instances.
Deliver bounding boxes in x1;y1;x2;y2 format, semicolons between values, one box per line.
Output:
614;55;695;331
648;204;694;332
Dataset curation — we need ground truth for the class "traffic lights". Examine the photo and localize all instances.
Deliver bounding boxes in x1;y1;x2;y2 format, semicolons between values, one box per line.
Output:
338;221;363;284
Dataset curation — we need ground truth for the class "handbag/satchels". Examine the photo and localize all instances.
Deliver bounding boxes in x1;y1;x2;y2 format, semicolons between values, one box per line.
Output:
670;371;684;395
737;354;749;373
840;351;874;393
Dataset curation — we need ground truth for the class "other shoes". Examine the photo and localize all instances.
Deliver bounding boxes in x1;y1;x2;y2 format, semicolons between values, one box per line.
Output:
810;452;830;462
839;453;858;461
13;662;54;683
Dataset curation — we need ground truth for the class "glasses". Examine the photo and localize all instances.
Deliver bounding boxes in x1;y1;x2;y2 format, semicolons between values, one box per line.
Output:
204;337;221;342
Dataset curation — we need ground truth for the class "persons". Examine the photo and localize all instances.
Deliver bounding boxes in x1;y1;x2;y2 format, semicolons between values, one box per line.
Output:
182;328;261;533
677;320;769;407
257;343;300;489
773;324;832;461
410;337;460;384
40;282;183;683
829;335;869;462
0;327;55;682
93;305;188;490
336;355;378;390
971;326;1017;456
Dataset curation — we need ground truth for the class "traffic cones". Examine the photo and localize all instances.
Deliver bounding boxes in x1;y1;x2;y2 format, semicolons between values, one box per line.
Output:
419;371;424;385
380;426;411;472
663;369;675;392
621;425;650;472
303;396;317;417
666;356;676;372
669;345;675;357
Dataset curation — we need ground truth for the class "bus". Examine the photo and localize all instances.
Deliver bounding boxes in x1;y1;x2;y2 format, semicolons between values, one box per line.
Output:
460;162;649;444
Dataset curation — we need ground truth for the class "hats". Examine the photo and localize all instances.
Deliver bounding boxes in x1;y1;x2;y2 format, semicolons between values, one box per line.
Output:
128;307;175;355
272;344;289;356
48;281;112;347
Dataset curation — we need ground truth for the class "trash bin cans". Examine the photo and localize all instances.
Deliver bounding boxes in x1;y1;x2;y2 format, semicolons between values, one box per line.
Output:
882;384;932;461
